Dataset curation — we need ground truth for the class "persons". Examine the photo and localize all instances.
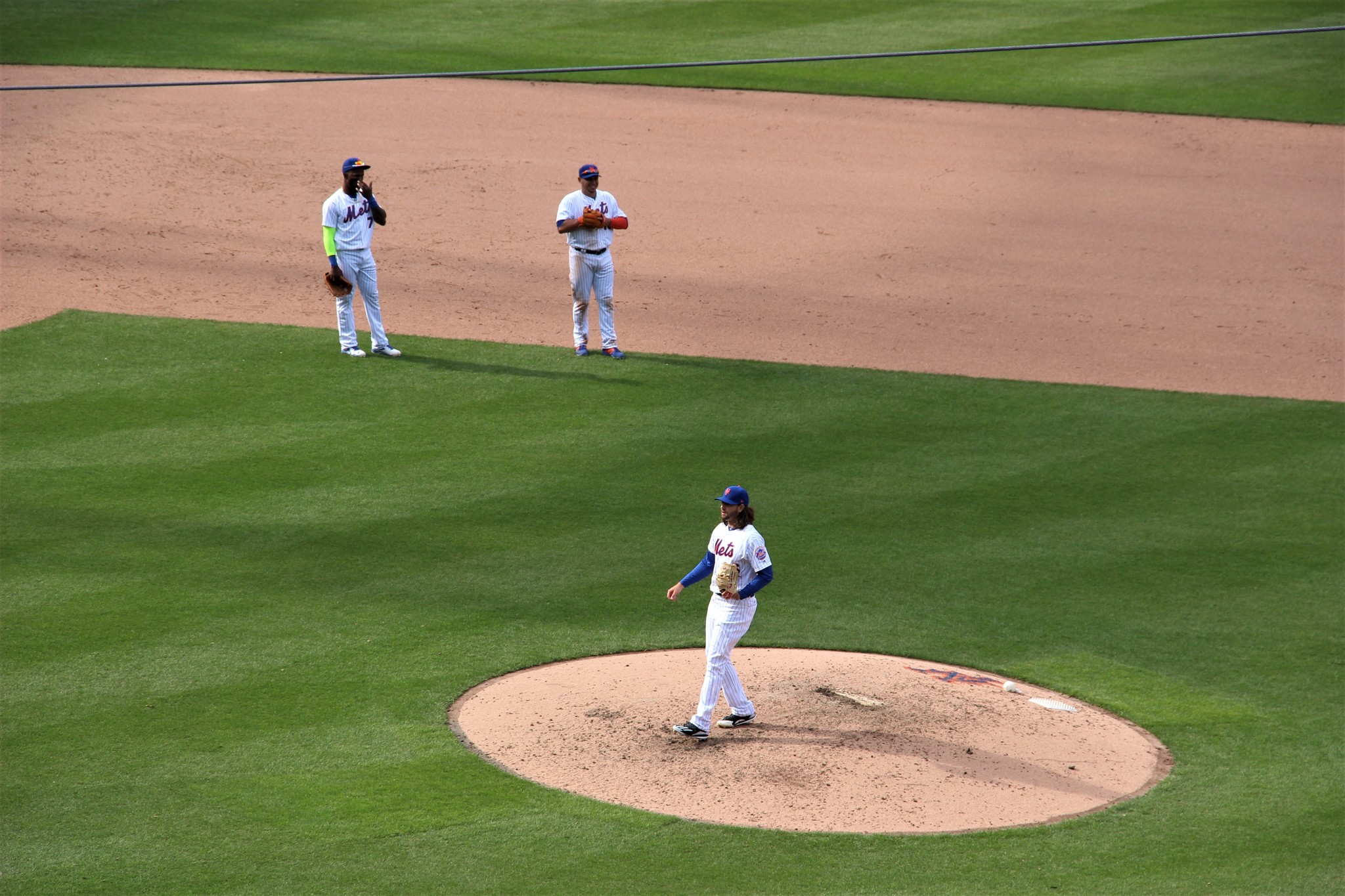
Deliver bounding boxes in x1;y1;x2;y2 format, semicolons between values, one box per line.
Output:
666;484;774;740
555;164;629;360
321;158;402;359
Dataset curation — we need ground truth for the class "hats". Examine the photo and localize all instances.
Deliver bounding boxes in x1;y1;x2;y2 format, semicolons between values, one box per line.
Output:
579;163;601;179
714;485;749;506
342;157;370;173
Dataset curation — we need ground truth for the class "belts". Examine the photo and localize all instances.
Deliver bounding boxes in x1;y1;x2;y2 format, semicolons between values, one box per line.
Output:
717;593;754;598
575;248;607;255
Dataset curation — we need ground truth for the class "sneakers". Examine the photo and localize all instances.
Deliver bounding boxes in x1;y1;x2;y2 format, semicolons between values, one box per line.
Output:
672;721;708;740
341;346;366;357
371;345;401;356
576;346;588;356
717;713;756;728
602;348;624;359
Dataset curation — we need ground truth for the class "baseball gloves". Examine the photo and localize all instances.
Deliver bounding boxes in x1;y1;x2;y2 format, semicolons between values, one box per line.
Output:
582;206;608;229
715;560;742;603
323;271;352;301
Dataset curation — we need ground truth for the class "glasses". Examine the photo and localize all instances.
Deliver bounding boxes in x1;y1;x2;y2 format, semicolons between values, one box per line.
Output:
343;161;365;168
580;167;599;176
